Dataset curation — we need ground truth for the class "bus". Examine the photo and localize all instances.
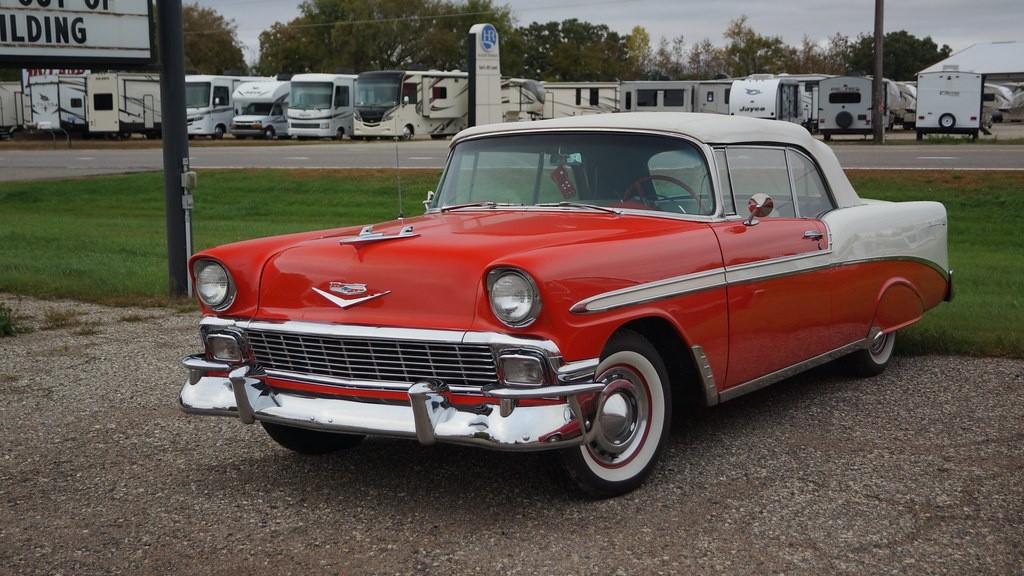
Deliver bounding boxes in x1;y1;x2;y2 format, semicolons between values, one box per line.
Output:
286;74;359;142
185;74;278;140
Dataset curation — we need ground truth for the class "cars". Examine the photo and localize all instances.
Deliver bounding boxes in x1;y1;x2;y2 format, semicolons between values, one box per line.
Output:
173;112;955;501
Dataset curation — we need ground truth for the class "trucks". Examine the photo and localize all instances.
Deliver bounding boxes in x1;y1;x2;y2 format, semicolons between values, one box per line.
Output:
87;72;161;140
229;80;295;140
27;75;88;140
541;82;622;119
352;70;547;143
620;70;1024;141
